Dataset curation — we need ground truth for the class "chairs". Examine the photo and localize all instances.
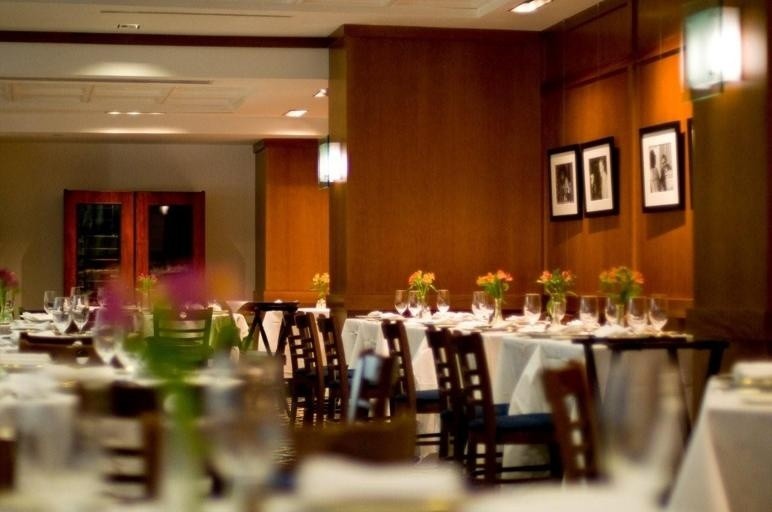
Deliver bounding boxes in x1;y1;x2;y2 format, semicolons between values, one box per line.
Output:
533;355;676;506
0;298;561;499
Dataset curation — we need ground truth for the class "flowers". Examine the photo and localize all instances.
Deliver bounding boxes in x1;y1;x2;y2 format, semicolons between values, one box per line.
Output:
597;260;648;308
472;268;515;299
312;270;331;299
533;266;583;296
404;267;444;300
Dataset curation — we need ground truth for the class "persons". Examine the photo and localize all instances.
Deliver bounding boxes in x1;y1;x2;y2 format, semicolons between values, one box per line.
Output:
556;150;674;203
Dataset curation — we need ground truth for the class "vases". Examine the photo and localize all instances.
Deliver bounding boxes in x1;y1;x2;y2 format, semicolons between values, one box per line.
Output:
546;292;568;317
316;298;329;312
615;291;633;323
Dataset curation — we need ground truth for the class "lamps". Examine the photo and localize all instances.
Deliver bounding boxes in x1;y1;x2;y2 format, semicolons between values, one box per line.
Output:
313;133;346;189
674;1;742;101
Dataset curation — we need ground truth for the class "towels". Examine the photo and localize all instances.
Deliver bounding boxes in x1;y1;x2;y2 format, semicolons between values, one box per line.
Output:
728;356;772;384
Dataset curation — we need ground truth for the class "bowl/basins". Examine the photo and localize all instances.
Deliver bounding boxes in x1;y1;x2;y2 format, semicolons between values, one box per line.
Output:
715;358;771;405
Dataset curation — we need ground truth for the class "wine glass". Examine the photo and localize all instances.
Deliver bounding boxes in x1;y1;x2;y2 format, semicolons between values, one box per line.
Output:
0;308;290;510
41;281;90;338
391;288;671;336
97;287;109;307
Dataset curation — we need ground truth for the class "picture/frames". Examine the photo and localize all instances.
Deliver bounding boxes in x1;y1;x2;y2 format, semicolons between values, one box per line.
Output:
581;135;618;218
635;121;685;213
545;144;584;222
